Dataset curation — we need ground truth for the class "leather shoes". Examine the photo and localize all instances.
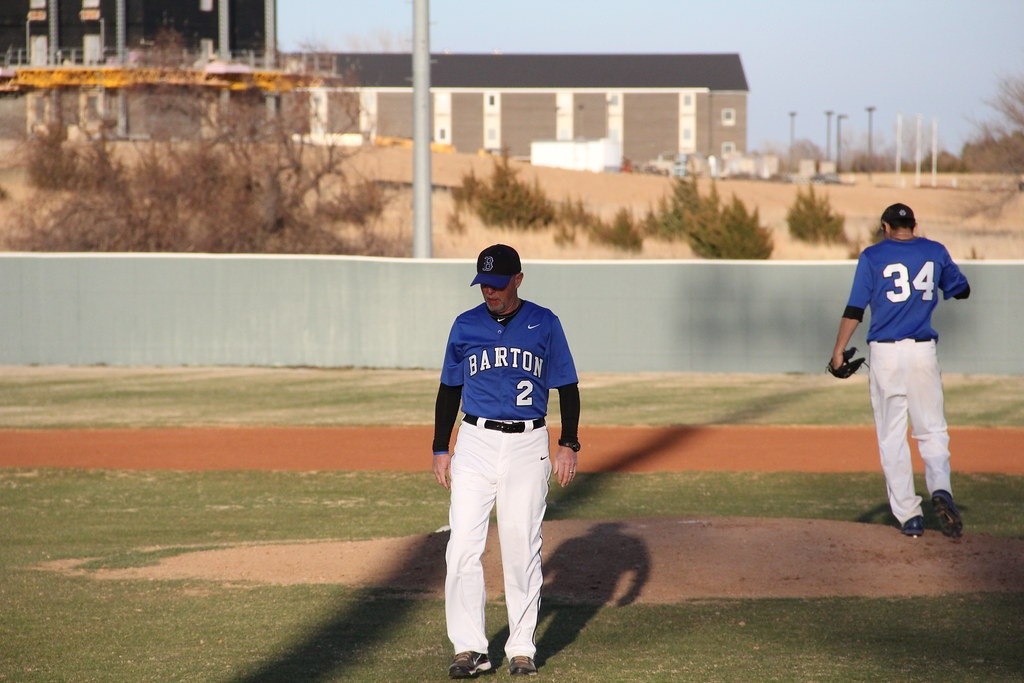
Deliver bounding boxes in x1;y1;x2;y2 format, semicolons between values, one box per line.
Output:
903;516;924;535
931;489;963;535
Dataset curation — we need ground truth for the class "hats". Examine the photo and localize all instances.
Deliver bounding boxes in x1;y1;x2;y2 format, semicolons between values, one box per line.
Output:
470;245;522;290
881;203;914;222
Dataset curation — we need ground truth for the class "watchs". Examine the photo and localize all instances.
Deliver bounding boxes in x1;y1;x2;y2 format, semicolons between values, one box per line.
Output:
558;439;580;452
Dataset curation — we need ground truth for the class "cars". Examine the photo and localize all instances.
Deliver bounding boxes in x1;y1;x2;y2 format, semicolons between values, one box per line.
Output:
640;146;780;182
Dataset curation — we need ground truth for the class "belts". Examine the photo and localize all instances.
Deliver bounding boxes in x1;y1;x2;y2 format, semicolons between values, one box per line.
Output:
462;414;545;433
877;339;931;343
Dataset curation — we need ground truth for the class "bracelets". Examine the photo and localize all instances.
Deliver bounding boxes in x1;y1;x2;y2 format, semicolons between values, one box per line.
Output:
433;451;449;455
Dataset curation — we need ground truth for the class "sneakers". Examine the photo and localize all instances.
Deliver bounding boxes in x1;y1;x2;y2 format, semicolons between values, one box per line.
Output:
448;651;491;677
509;656;537;675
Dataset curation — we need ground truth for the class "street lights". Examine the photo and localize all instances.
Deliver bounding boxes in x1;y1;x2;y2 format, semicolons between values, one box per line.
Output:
787;102;879;178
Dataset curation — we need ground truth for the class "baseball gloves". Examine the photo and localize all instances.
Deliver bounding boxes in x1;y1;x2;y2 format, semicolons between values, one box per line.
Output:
826;346;870;379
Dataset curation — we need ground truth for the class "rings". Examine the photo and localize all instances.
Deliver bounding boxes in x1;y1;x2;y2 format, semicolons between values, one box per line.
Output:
570;471;573;473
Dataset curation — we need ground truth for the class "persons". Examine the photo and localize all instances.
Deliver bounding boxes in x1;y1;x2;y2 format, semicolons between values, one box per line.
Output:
433;244;581;677
829;203;970;538
